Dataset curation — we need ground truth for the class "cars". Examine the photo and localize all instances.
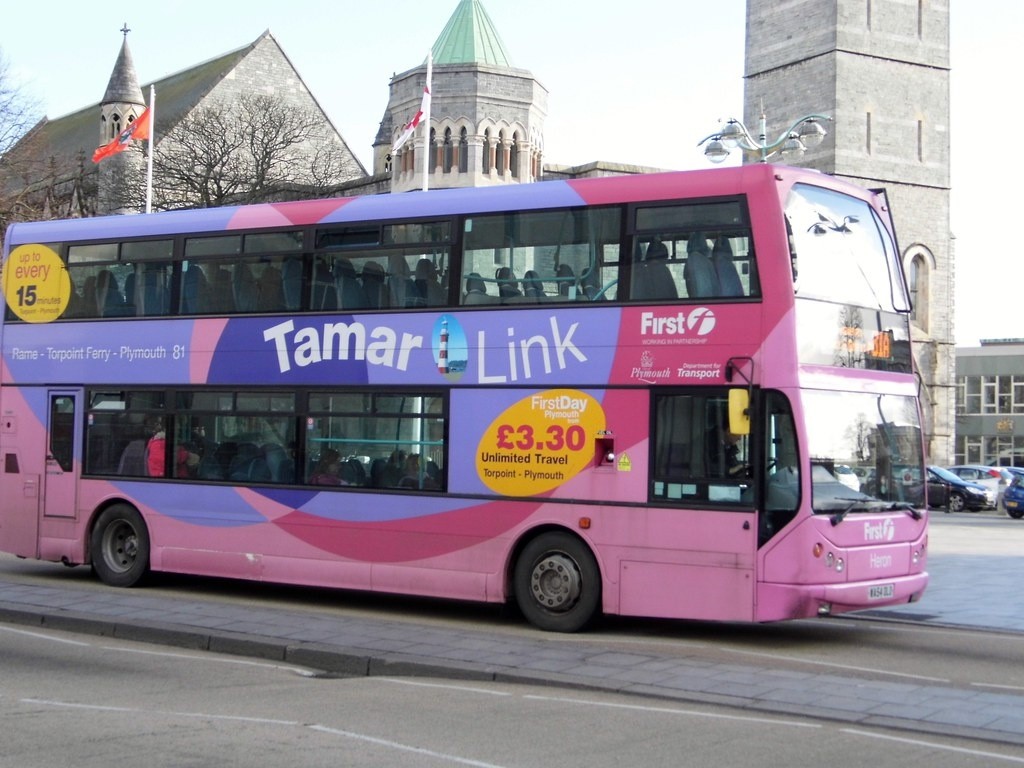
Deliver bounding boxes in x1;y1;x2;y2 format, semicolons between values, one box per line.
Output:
925;465;1016;497
831;463;877;494
1005;467;1024;478
867;463;997;512
1002;473;1024;518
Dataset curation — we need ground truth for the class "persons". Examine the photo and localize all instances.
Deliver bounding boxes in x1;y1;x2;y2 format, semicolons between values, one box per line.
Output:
715;422;741;468
143;419;444;490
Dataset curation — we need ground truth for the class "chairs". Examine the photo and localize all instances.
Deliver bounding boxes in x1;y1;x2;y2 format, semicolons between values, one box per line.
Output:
55;412;443;492
62;232;747;297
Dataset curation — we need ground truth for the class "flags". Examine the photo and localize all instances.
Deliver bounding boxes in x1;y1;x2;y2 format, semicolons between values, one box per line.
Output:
393;82;430;152
91;108;150;164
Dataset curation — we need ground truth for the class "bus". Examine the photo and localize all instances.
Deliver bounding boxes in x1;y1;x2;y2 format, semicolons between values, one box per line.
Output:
0;165;932;633
